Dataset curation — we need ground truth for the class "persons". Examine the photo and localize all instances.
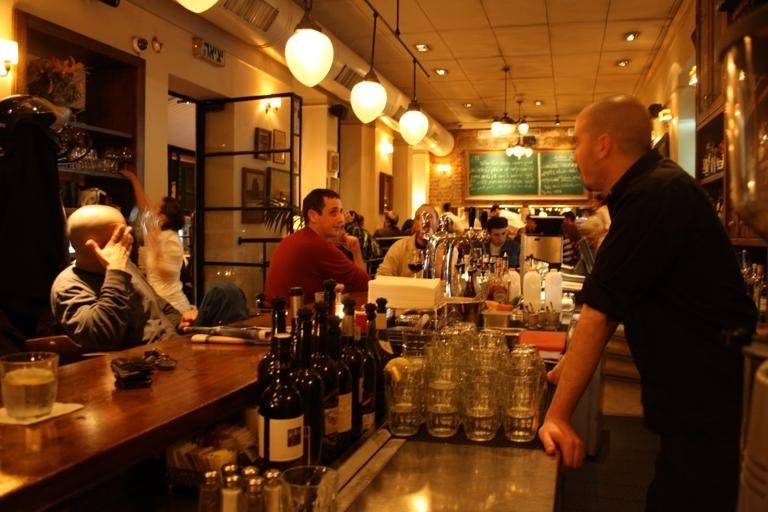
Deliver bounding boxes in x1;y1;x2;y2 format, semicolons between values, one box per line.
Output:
119;169;190;315
263;188;370;317
343;192;611;282
48;204;250;351
536;93;758;510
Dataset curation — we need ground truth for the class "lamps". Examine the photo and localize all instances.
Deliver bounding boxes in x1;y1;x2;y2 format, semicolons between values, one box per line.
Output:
285;1;430;145
491;64;533;159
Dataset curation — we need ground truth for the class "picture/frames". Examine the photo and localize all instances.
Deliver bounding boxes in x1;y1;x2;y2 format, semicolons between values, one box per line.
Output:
241;128;340;223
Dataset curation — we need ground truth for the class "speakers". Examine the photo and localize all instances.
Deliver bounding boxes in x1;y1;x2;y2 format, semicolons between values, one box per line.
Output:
329;104;349;120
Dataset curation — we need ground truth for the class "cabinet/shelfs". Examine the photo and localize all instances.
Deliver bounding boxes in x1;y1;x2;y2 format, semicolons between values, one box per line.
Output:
695;0;768;450
56;122;134;178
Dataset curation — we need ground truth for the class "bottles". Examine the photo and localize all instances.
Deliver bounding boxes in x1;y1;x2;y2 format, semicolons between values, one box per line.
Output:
699;132;725;179
64;179;79;207
255;280;396;471
735;248;767;323
417;211;511;304
196;463;288;512
513;206;560;216
504;267;583;354
707;195;723;224
101;190;112;205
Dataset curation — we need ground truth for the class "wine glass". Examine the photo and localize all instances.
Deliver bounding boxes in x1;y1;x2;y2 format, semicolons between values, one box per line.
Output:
56;132;134;175
406;250;430;280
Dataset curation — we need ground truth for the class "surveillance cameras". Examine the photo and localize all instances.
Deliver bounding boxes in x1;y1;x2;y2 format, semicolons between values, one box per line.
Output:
133;37;150;52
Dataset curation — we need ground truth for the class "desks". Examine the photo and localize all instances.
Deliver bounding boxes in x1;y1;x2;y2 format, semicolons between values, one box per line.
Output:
0;284;367;500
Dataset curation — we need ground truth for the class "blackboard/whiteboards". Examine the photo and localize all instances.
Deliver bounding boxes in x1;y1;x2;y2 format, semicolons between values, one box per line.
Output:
463;147;591;202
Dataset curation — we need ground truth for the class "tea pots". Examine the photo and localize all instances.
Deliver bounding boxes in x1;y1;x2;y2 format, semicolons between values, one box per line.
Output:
576;236;599;275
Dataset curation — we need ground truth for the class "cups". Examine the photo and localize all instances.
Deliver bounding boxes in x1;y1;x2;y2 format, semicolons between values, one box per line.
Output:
385;319;548;442
75;187;106;209
279;465;342;512
2;350;60;420
